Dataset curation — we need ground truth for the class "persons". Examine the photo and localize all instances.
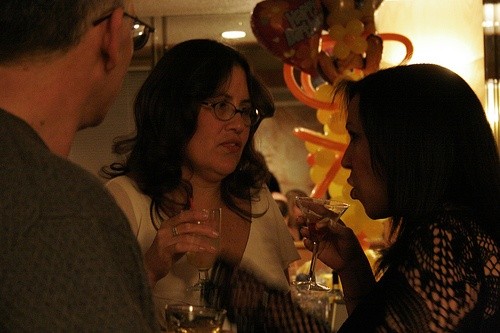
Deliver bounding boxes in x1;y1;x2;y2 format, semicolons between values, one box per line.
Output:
297;65;500;333
0;0;155;333
97;39;312;333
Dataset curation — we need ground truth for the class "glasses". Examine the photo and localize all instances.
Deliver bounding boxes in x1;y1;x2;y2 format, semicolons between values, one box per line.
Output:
93;11;155;50
203;100;261;126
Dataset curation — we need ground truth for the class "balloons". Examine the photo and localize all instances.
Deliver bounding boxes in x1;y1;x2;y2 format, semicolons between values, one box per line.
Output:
251;0;413;281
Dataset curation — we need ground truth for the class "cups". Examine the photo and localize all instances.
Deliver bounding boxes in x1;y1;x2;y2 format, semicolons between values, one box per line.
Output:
164;305;226;333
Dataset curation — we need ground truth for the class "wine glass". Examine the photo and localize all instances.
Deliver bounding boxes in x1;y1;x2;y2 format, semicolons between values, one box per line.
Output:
292;200;348;291
187;207;222;288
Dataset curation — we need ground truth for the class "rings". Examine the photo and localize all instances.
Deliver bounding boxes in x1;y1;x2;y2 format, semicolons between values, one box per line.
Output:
173;227;178;235
